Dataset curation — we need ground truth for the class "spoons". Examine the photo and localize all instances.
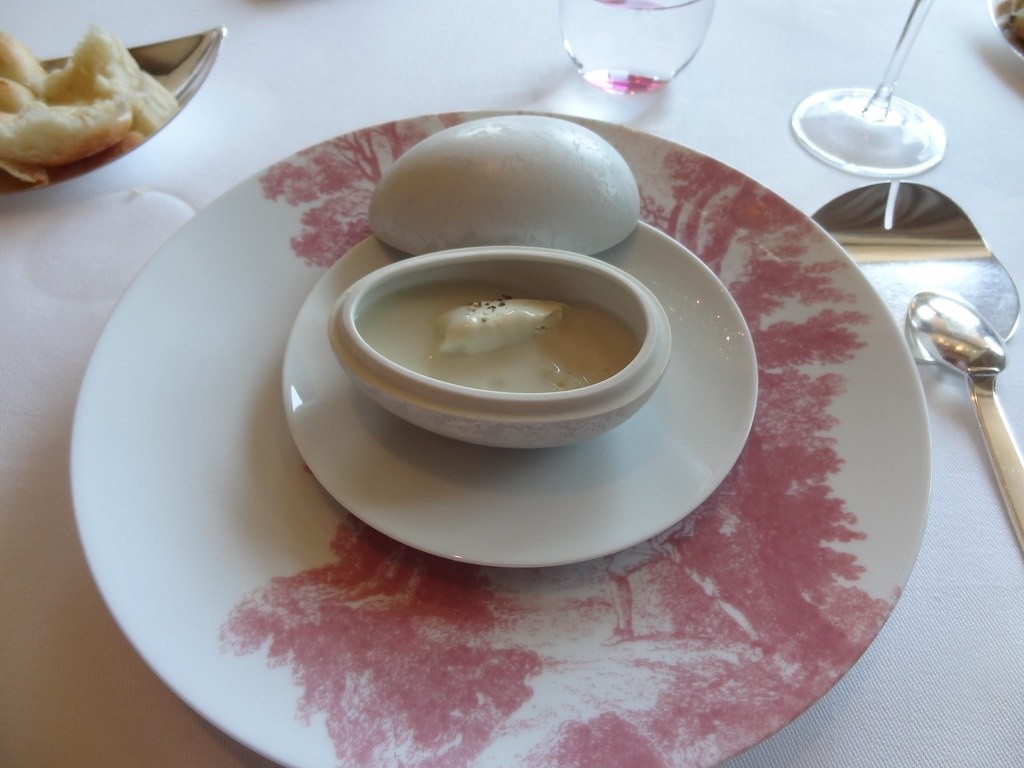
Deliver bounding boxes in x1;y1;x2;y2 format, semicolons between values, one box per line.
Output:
909;289;1024;556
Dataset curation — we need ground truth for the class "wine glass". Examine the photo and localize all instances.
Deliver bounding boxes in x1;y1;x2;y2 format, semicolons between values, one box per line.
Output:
791;0;947;176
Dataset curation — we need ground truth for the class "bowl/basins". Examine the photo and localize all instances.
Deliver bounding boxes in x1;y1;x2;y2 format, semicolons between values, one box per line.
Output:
329;245;672;451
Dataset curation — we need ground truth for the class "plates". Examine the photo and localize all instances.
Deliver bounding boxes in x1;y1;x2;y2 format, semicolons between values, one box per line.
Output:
280;220;761;567
64;109;933;767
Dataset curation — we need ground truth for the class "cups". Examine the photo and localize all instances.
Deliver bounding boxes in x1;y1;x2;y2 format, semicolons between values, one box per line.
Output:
562;0;717;97
